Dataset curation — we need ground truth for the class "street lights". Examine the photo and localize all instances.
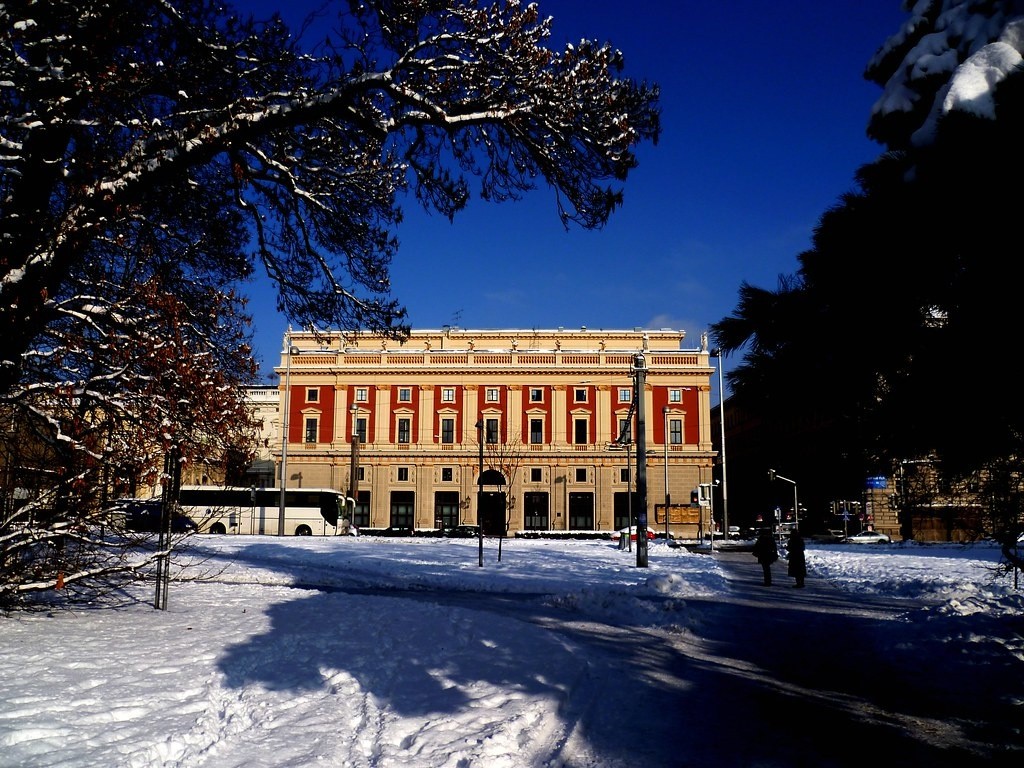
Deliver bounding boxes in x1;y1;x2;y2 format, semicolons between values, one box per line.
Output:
661;405;672;541
276;344;301;538
708;345;730;543
474;418;484;567
348;401;359;526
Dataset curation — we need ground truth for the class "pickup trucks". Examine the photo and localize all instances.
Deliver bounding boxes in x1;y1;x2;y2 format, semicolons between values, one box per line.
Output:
811;528;846;543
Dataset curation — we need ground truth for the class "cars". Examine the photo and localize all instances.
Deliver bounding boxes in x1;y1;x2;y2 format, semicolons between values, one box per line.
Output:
609;524;655;542
845;530;890;545
728;525;741;539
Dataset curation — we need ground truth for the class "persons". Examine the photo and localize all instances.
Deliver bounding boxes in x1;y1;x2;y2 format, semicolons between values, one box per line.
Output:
755;530;778;587
785;529;807;589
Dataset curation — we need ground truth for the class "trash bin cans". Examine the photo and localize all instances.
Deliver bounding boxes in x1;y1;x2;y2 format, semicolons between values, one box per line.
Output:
720;519;729;532
12;498;30;522
619;532;630;550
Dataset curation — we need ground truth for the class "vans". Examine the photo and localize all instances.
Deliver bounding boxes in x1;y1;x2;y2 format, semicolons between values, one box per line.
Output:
126;503;199;536
376;523;415;537
438;525;486;538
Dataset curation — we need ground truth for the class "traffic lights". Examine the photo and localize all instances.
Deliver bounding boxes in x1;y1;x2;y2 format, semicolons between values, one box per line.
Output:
691;490;698;503
859;514;864;521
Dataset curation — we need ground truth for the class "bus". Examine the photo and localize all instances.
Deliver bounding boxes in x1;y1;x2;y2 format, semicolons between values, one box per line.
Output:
178;485;356;538
